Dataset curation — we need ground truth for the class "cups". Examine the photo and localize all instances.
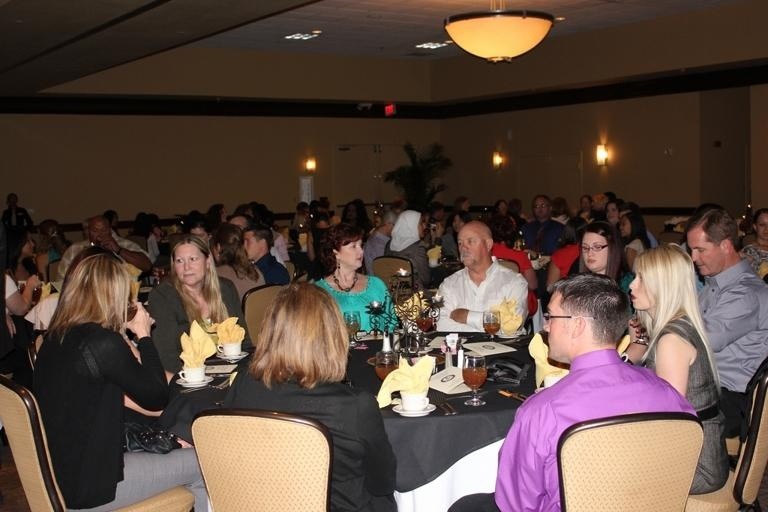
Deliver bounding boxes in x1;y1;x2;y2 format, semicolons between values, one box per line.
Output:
399;391;430;410
180;368;205;383
429;257;438;265
217;345;241;356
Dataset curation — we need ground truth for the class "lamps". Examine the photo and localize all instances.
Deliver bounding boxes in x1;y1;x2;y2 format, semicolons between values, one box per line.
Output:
303;155;318;176
443;0;554;64
597;144;606;165
493;152;500;169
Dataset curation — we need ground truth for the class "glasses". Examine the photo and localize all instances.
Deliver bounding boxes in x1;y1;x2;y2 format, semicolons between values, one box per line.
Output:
581;244;609;253
542;313;598;325
533;202;553;209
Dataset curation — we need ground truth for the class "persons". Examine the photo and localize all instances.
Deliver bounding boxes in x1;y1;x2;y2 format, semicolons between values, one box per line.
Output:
621;245;729;496
686;202;768;438
1;193;767;368
448;271;702;512
32;251;211;512
222;283;397;512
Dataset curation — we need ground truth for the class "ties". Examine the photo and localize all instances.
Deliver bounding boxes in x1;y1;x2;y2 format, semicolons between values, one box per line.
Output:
531;226;544;253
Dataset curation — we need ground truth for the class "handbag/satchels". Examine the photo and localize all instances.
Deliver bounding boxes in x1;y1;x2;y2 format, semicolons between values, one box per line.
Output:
122;421;182;454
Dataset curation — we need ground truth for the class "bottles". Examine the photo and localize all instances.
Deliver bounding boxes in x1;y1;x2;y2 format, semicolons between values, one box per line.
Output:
457;345;464;368
393;333;400;350
462;356;487;407
382;327;392;353
445;347;453;368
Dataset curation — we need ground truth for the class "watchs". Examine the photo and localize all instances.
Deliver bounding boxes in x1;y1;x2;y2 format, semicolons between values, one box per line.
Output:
632;337;649;349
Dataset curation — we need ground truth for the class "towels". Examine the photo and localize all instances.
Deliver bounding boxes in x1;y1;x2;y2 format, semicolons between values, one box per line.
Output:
374;353;437;409
528;333;570;389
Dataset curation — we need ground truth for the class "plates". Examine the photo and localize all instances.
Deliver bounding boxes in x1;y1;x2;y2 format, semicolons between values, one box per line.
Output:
393;402;436;418
216;352;249;360
176;375;214;390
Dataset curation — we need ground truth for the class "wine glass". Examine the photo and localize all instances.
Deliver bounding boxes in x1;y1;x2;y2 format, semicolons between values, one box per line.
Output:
483;310;501;342
126;304;148;343
415;309;434;347
343;312;361;347
32;285;42;305
375;352;399;398
153;278;160;288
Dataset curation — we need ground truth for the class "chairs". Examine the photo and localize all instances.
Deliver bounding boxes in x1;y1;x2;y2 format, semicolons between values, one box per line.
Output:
556;411;704;512
0;374;196;512
684;375;768;512
190;408;334;512
722;356;768;456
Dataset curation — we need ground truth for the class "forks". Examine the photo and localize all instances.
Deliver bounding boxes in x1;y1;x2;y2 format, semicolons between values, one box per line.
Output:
430;391;458;416
